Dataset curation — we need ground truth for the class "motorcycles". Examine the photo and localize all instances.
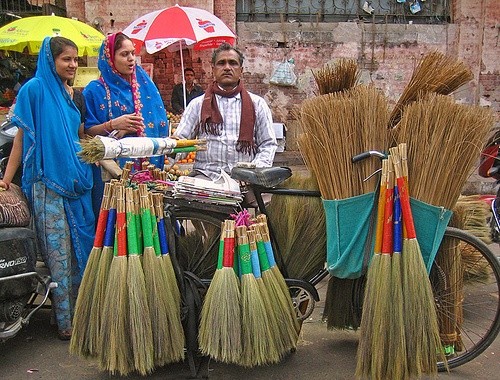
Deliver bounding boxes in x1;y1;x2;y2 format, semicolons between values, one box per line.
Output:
0;178;52;344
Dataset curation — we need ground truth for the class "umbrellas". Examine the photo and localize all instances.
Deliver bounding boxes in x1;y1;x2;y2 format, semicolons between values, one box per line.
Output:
122;3;239;111
0;13;108;58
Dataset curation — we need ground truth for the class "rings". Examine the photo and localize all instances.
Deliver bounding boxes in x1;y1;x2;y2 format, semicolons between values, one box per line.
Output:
130;123;133;127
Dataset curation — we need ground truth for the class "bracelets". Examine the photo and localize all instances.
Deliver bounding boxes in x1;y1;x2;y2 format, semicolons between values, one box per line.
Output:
104;122;109;131
109;119;114;130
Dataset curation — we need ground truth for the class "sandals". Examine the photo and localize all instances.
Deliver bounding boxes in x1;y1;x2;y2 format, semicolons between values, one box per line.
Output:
57;325;73;341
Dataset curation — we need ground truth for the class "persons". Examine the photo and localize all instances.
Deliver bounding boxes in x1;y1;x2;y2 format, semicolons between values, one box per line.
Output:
164;43;278;236
0;36;120;341
171;67;205;114
81;31;170;234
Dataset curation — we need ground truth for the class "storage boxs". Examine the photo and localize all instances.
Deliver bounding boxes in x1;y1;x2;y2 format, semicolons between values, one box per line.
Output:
71;66;102;91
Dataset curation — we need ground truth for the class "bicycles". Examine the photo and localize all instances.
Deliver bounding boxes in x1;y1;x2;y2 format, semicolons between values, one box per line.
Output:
129;152;500;377
